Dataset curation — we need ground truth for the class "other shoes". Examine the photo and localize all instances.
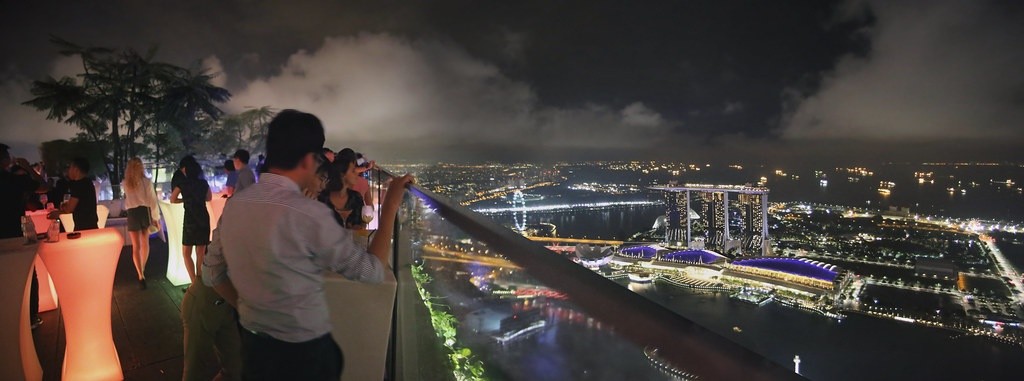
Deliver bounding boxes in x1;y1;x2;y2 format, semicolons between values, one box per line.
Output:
182;286;188;293
30;316;44;330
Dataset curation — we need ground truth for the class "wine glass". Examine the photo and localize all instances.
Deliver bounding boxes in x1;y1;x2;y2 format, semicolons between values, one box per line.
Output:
40;194;47;211
336;208;352;228
361;205;374;230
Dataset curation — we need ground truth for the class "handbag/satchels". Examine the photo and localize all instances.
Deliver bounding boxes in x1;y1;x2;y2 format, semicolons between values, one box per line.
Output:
148;225;158;235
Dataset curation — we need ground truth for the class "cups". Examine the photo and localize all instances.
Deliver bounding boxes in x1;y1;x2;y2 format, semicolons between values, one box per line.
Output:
47;220;60;242
63;194;71;203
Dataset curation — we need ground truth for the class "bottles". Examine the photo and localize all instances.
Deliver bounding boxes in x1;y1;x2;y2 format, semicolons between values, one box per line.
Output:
20;214;38;243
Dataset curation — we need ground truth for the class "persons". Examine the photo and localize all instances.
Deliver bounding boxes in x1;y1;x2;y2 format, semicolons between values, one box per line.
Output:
171;156;195;193
223;160;239;196
48;158;98;231
123;157;160;287
0;143;44;239
169;163;212;291
300;148;375;230
233;150;255;193
201;110;415;381
